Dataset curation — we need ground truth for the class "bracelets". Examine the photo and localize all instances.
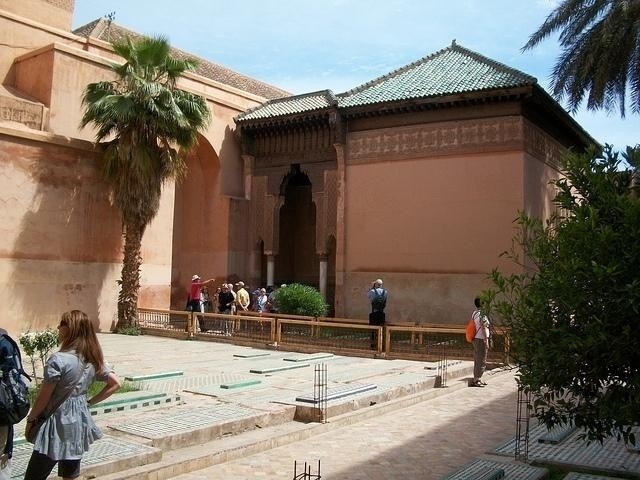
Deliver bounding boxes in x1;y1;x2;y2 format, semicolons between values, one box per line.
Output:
27;420;35;423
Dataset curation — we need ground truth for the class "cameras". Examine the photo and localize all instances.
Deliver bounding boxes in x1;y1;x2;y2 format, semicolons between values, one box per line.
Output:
373;283;376;287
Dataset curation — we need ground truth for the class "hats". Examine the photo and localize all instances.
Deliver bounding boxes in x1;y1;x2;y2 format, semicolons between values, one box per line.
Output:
375;279;382;285
216;281;245;290
200;285;209;290
192;275;200;281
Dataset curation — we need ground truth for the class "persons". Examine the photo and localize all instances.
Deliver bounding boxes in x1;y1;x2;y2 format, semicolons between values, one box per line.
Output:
185;274;282;334
25;310;121;480
0;329;22;480
367;278;389;350
471;297;491;387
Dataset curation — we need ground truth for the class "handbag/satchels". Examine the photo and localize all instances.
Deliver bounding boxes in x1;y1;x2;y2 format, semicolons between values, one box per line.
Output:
25;417;44;443
186;303;197;311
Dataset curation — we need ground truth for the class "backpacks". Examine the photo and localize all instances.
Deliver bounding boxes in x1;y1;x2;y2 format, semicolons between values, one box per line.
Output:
466;309;486;343
372;288;386;310
1;332;30;427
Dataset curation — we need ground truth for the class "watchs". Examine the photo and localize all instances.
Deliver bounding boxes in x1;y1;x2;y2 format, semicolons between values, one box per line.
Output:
88;400;90;407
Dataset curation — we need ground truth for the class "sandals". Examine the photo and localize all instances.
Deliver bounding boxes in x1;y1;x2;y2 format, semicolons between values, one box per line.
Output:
471;380;487;387
